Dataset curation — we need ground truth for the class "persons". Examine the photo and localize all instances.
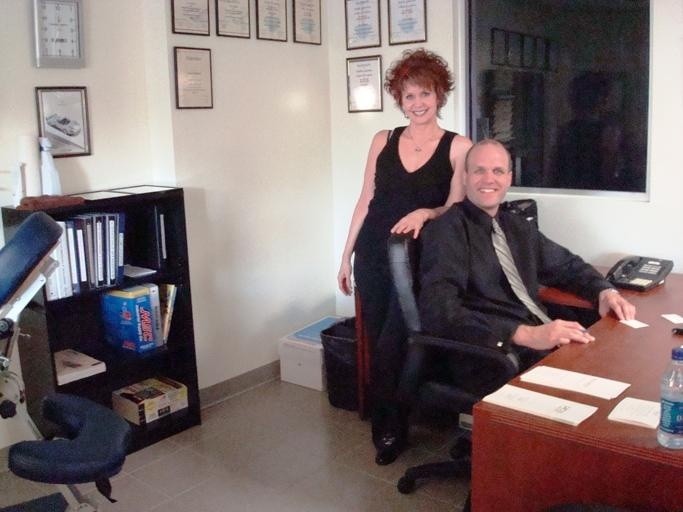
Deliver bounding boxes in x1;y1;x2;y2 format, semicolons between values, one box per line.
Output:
340;46;471;464
418;141;635;375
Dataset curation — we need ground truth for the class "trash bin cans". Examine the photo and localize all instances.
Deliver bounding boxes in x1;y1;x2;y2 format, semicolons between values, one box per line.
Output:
320;316;360;410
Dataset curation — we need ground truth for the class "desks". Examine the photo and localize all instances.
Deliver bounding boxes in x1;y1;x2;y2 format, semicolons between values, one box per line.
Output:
472;263;683;512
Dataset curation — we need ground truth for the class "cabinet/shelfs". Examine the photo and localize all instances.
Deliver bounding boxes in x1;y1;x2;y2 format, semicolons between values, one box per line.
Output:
481;69;544;185
0;183;201;458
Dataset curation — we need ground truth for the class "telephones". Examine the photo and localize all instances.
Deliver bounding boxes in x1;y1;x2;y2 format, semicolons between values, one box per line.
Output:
605;256;673;292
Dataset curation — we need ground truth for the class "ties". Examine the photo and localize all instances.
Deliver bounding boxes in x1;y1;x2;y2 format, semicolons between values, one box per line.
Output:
492;219;550;324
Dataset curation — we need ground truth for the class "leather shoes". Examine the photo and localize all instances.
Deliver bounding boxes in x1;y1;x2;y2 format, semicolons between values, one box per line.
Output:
372;430;407;466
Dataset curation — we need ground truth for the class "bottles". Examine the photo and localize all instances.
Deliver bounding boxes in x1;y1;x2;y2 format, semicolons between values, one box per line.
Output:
657;347;683;449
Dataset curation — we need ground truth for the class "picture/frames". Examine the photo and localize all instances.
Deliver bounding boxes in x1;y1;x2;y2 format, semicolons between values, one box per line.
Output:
344;0;381;51
345;55;383;114
34;86;92;158
173;45;213;109
257;0;288;41
387;0;427;46
293;0;322;45
216;0;251;39
34;1;86;70
172;0;211;36
490;26;560;73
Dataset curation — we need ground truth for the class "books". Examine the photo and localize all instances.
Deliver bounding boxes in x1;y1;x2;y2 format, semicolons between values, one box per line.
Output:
110;377;190;424
142;281;163;348
53;350;106;385
39;212;129;302
101;288;155;351
159;287;179;343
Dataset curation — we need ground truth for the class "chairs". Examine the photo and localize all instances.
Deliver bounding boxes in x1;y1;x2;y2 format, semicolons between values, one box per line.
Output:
386;229;518;512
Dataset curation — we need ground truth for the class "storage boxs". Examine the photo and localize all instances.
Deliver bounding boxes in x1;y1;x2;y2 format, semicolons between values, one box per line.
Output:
280;315;345;393
